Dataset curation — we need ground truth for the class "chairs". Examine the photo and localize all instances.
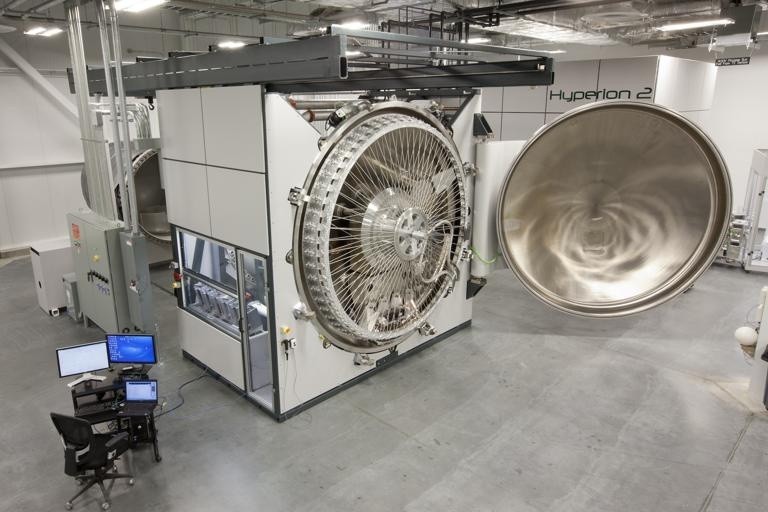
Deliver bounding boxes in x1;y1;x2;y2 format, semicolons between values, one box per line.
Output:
48;412;134;512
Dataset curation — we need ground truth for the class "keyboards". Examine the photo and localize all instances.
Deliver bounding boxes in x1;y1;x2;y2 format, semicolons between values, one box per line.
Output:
74;401;114;417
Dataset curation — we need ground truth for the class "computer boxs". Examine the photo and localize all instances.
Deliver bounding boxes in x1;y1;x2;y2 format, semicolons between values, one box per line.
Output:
131;415;149;440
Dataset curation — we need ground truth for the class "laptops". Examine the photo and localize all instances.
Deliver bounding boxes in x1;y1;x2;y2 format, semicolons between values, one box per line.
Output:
117;379;158;417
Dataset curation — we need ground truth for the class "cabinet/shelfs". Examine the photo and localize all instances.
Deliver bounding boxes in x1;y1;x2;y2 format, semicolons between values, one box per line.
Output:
70;409;162;463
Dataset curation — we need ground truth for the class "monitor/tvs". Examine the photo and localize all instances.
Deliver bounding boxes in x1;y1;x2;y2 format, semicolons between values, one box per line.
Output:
55;339;109;388
103;333;158;366
